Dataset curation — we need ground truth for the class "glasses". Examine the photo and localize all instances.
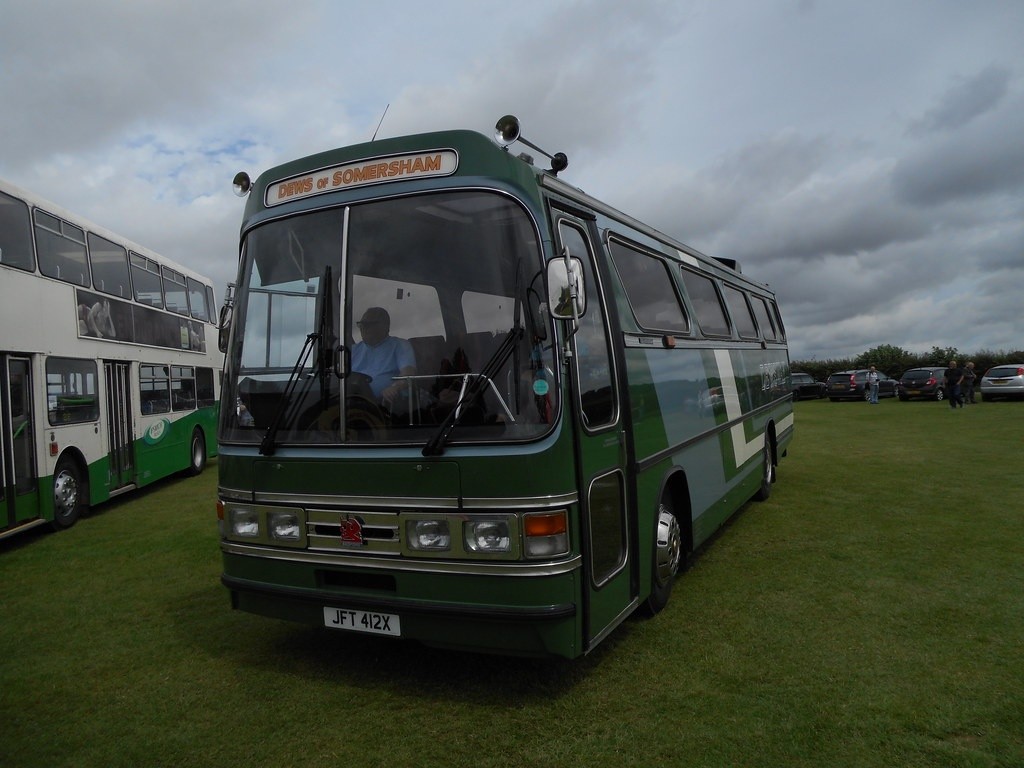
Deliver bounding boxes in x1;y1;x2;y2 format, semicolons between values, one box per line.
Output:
356;322;384;329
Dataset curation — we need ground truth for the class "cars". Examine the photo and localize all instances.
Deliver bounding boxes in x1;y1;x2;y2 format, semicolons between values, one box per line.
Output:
782;376;790;393
770;378;783;395
700;385;726;415
980;364;1024;401
791;372;827;401
826;370;900;401
898;367;979;402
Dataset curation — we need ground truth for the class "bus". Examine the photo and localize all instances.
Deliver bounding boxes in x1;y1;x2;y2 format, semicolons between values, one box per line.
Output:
0;192;219;534
212;115;797;665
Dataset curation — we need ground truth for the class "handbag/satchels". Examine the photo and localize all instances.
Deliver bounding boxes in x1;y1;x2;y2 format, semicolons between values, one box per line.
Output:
864;383;870;390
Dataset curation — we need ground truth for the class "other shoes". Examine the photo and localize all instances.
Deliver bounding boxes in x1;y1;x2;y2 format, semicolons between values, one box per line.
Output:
952;406;956;409
960;399;963;408
870;402;879;404
973;401;978;404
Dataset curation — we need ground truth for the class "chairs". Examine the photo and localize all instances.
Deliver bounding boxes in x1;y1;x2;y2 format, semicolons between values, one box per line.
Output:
0;246;203;423
407;327;547;427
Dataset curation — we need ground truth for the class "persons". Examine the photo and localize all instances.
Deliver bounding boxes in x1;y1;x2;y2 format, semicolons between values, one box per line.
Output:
76;298;117;338
187;320;205;353
350;307;417;413
943;361;978;408
866;366;880;405
